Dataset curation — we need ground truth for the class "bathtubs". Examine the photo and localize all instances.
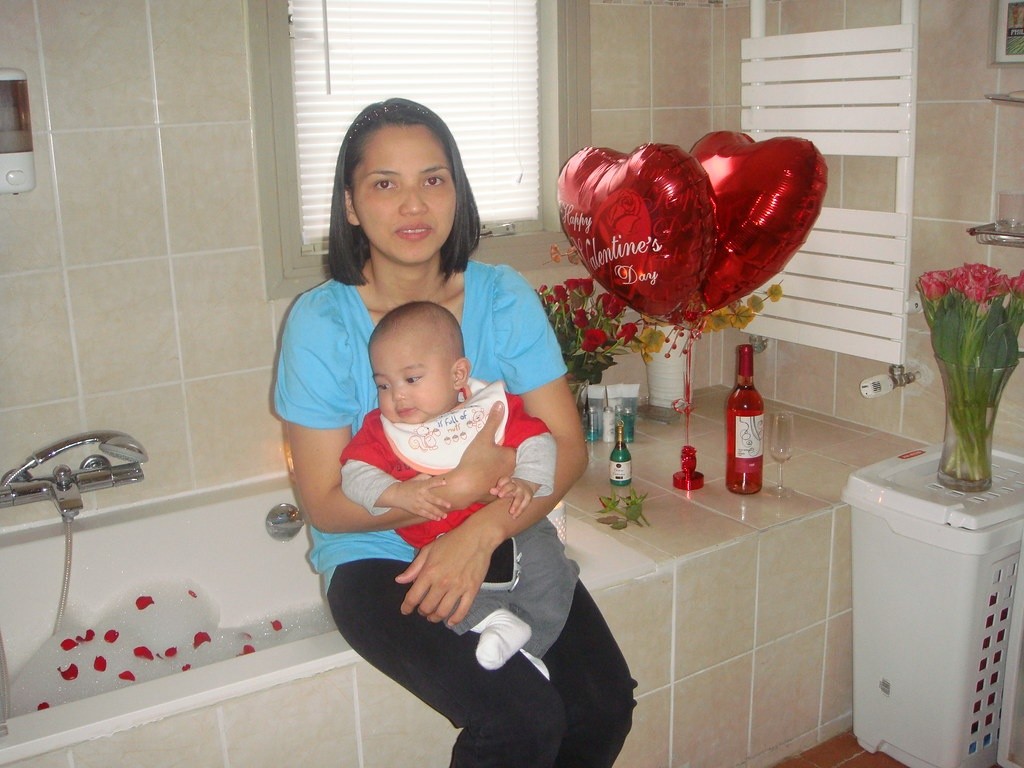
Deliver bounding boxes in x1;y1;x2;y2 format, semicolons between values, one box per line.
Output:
0;470;463;768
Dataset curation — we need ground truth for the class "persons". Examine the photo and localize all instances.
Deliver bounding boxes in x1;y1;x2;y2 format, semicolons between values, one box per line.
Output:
265;90;643;768
336;298;581;684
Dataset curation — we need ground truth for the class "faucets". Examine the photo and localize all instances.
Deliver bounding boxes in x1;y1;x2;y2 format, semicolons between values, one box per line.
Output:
45;476;85;525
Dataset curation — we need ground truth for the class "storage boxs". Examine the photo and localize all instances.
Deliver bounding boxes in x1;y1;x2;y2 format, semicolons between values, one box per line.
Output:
837;441;1024;768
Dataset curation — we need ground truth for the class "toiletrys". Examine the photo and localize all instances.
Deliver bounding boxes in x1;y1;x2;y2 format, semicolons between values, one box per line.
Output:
585;383;641;487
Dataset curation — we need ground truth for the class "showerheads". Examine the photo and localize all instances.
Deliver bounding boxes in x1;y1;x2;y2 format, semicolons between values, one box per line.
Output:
26;429;151;464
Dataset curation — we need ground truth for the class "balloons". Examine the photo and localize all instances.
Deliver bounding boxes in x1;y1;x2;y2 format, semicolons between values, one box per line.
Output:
685;128;832;319
553;128;717;345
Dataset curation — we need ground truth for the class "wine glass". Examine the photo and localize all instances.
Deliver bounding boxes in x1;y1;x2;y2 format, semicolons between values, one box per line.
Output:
765;411;794;498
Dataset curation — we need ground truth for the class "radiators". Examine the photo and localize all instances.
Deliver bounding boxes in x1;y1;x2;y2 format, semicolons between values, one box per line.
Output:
733;0;922;368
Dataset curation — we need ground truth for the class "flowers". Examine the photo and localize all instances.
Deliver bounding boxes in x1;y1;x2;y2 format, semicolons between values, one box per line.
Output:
532;278;639;383
912;262;1024;370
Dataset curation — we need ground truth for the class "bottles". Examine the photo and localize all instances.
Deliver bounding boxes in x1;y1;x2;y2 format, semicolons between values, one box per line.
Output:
727;344;765;494
609;420;632;486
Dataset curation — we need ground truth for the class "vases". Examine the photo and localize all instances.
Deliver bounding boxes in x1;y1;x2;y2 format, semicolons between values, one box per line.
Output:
933;355;1018;495
564;383;587;412
